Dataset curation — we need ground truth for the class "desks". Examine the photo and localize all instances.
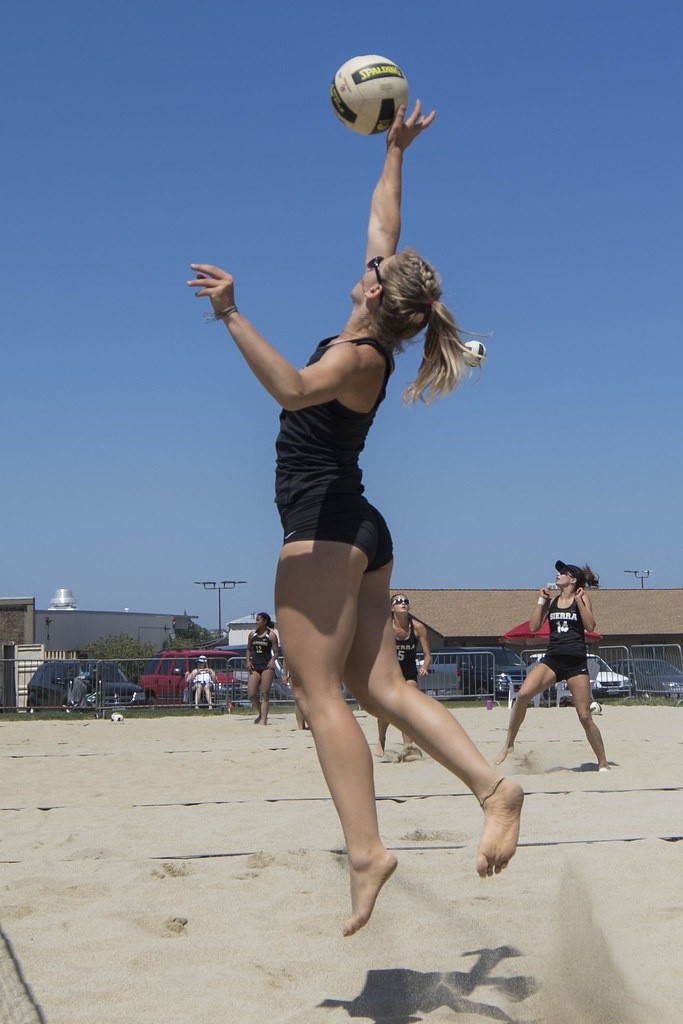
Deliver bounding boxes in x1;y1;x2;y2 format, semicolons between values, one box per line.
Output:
507;683;540;709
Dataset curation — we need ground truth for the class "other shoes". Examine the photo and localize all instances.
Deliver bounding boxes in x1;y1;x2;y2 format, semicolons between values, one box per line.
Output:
63;704;71;714
68;701;78;707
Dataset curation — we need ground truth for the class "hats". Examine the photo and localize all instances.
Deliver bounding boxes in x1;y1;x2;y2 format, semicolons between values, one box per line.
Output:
79;652;87;659
555;559;585;586
197;656;208;663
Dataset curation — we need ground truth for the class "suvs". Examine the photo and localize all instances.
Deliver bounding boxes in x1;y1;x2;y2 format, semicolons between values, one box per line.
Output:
608;659;683;699
26;659;146;713
531;654;635;698
138;645;352;706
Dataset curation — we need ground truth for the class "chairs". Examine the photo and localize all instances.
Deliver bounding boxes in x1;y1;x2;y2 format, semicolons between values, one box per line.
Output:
66;679;101;712
185;671;220;705
555;679;595;708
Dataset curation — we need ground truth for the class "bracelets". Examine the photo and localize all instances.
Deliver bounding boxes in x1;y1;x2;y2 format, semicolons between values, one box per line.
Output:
538;597;546;605
202;304;237;323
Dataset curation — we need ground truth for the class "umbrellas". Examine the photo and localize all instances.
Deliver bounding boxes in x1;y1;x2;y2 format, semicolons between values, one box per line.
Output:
499;616;602;644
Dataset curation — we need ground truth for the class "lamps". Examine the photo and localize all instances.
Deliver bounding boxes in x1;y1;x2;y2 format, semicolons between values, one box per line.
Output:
47;619;54;626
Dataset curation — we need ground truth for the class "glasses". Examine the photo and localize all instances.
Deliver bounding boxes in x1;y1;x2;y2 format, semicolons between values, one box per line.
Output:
558;569;574;579
392;599;409;607
368;255;385;303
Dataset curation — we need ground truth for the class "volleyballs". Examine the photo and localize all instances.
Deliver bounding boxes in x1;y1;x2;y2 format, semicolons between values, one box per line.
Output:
589;702;602;715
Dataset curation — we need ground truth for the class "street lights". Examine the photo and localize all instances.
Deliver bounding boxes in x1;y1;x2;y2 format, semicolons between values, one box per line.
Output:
46;617;54;660
625;570;653;589
195;581;247;639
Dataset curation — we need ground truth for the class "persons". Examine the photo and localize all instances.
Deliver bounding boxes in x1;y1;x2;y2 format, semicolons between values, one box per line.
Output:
187;99;525;938
187;655;217;709
282;657;306;730
495;560;610;772
374;593;432;761
69;652;96;693
246;612;278;725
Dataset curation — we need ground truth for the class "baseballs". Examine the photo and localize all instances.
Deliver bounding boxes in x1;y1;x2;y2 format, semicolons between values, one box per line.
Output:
329;54;411;134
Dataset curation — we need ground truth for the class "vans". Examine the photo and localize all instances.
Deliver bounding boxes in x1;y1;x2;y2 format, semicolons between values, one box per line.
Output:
415;648;527;700
